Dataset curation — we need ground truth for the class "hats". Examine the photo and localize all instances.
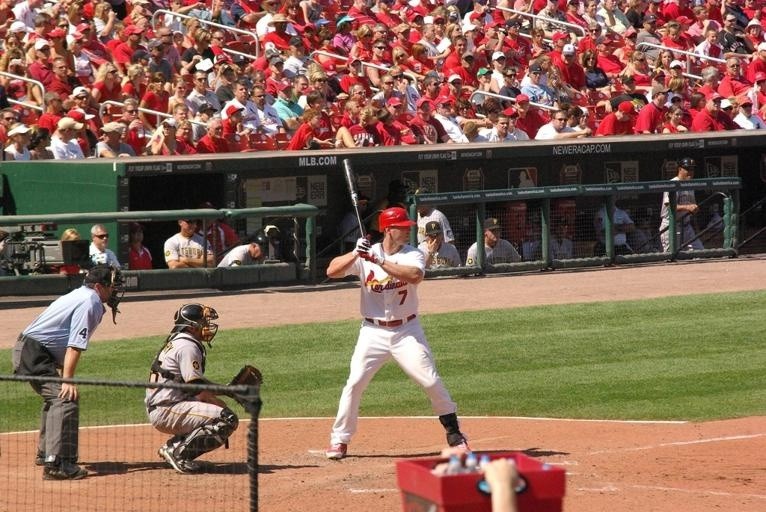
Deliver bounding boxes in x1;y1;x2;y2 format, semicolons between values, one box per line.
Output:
617;60;682;116
6;103;243;136
483;217;504;230
745;19;761;33
447;51;541;82
10;21;163;61
213;13;360;91
552;14;656;56
72;87;88;97
425;221;441;235
386;96;452;107
503;94;529;117
396;12;519;33
705;72;766;108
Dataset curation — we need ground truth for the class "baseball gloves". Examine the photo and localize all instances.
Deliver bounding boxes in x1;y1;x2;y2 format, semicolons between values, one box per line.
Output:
228;366;263;386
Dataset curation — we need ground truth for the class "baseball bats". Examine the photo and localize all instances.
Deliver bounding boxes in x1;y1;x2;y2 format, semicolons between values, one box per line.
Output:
342;159;366;239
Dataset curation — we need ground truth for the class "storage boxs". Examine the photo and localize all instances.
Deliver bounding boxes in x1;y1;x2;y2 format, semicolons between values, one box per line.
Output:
393;446;566;512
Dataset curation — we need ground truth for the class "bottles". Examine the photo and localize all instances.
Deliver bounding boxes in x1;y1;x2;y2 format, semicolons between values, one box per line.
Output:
443;451;517;475
136;123;144;139
542;462;552;470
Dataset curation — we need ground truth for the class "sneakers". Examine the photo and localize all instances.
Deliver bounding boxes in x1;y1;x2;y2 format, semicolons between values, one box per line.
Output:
449;442;470;452
157;444;199;474
35;455;87;481
325;443;346;459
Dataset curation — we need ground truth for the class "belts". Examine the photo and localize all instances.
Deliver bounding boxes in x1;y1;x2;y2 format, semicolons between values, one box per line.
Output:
365;314;416;327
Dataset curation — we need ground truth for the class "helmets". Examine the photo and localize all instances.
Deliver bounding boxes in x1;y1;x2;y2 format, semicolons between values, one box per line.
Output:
680;157;697;166
170;304;218;348
84;263;125;313
378;207;416;232
255;235;268;251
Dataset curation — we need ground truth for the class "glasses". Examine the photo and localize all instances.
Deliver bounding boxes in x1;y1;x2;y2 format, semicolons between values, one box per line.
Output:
94;234;107;238
364;31;403;84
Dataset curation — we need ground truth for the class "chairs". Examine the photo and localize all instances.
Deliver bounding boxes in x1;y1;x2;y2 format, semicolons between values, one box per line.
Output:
0;0;765;160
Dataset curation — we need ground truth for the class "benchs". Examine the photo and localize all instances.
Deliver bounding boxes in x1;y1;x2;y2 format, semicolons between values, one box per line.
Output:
456;218;765;257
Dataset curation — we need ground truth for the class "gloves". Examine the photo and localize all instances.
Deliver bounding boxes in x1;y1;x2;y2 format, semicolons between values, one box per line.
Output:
353;234;383;265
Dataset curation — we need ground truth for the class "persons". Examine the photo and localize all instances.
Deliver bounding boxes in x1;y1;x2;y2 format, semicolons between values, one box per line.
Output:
410;188;463;268
60;224;121;266
325;205;470;463
478;454;521;511
129;201;268;267
465;217;522;264
7;263;123;482
339;178;410;255
550;215;573;261
658;157;705;260
595;196;657;262
0;0;766;162
143;300;240;476
